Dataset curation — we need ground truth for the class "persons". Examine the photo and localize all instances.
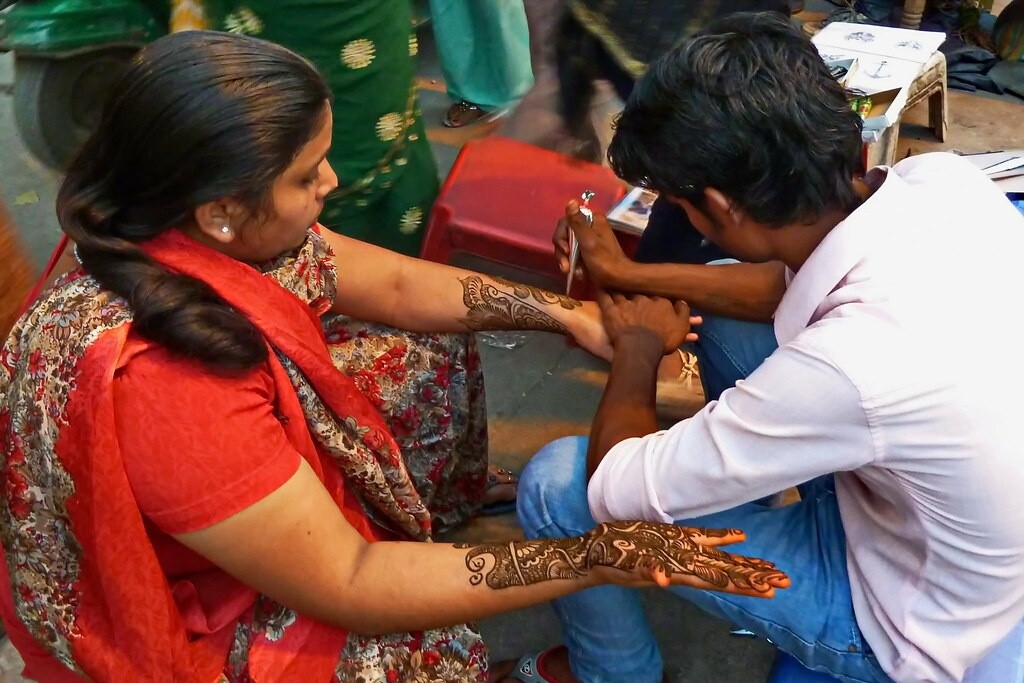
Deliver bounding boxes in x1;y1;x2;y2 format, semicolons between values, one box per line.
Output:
487;20;1024;683
0;29;790;683
201;0;965;261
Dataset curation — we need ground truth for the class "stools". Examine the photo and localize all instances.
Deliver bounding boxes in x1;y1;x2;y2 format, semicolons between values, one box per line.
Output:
419;135;641;348
866;50;947;173
767;650;843;683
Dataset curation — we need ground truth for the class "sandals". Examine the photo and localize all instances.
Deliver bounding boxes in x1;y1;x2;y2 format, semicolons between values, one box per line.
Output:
470;463;523;517
441;100;484;128
503;644;579;683
